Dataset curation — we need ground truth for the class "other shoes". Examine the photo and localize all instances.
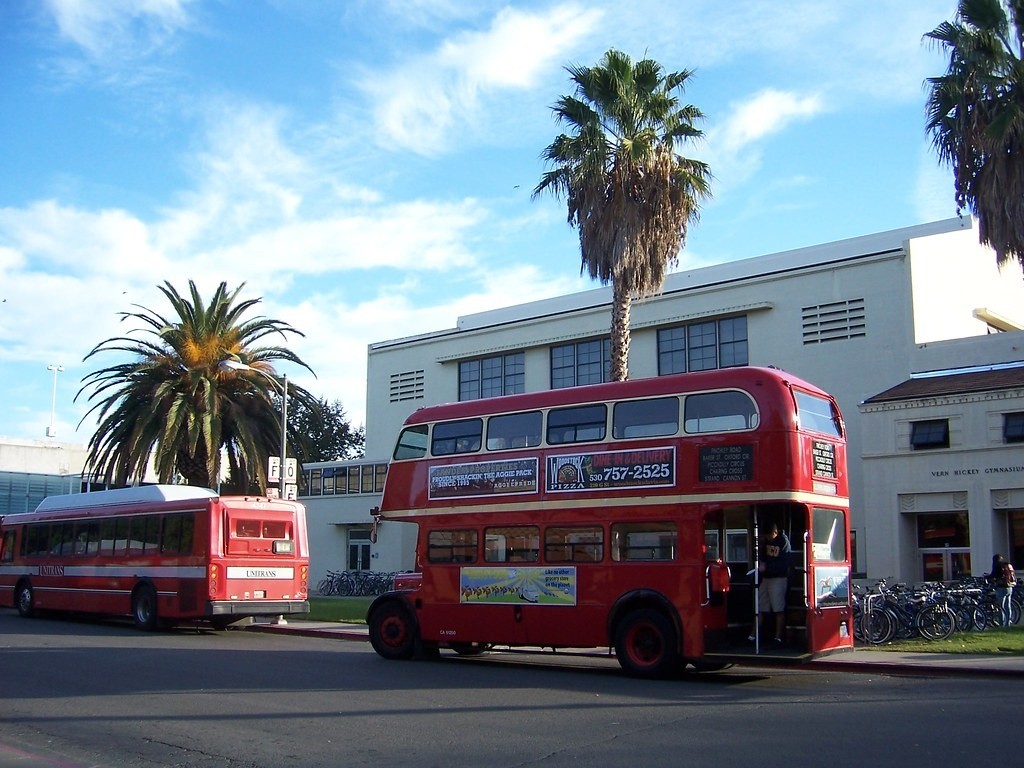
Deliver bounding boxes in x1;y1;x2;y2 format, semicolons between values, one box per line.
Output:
766;638;783;651
735;636;755;647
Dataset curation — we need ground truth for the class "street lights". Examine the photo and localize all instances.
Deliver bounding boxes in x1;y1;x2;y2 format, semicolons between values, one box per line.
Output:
218;359;289;498
46;365;64;425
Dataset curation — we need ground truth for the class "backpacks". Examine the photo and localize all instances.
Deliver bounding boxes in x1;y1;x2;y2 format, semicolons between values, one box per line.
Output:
998;562;1017;588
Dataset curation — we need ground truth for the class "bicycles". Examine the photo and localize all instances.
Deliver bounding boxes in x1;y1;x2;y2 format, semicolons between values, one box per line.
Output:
848;572;1024;643
318;567;415;597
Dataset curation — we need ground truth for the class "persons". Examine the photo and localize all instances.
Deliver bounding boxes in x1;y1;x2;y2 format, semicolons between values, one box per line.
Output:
818;576;833;595
984;555;1012;628
748;523;791;643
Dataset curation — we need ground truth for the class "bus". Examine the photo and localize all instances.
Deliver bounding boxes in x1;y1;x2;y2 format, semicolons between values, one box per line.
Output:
362;366;855;676
0;484;310;631
